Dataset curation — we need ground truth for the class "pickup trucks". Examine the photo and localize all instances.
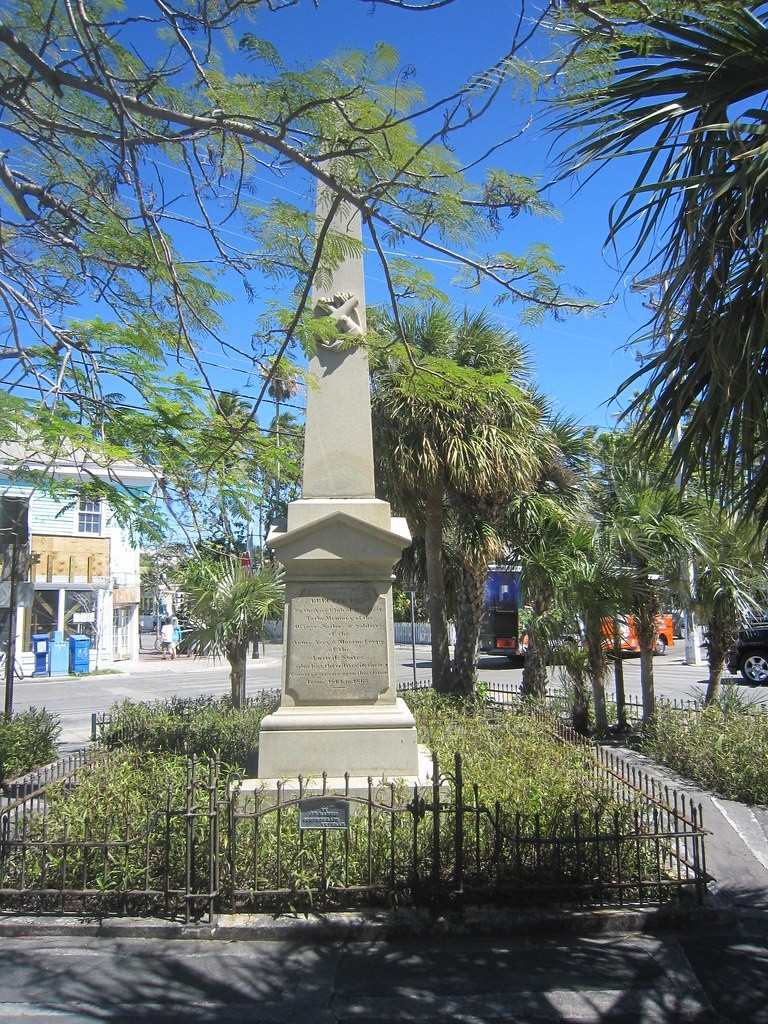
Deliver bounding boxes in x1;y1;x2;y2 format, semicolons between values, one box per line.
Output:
726;608;768;687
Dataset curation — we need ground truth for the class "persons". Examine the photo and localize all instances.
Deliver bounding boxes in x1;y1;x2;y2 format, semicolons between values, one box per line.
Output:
160;617;182;660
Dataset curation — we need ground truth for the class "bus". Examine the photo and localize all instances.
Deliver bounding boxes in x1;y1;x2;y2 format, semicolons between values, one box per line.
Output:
482;564;676;667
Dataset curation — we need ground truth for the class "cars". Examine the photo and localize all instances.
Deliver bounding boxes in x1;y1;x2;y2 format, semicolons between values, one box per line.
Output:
672;607;688;639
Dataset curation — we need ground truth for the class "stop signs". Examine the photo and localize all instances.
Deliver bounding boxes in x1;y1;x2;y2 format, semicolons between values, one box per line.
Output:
239;552;252;578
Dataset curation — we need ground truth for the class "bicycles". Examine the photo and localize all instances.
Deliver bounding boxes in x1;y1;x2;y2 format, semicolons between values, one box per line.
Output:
153;633;180;653
0;635;26;681
